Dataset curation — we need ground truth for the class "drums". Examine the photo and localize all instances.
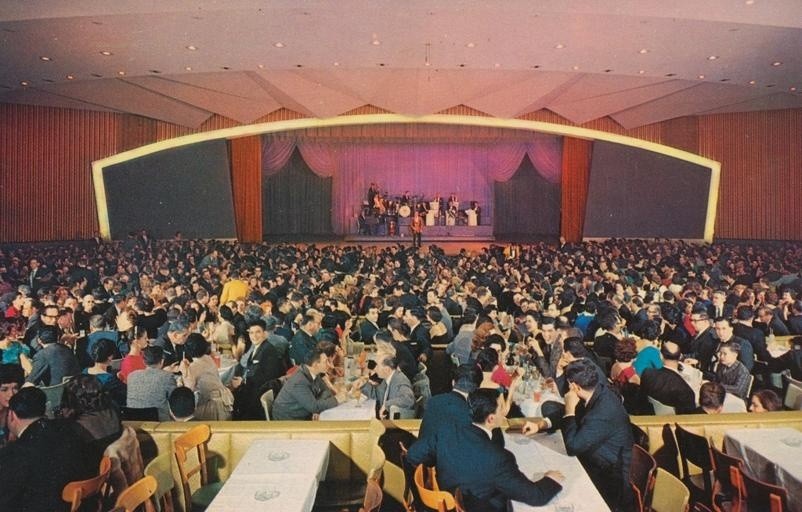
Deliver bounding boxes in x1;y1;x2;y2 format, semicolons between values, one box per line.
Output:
387;199;414;213
398;206;411;217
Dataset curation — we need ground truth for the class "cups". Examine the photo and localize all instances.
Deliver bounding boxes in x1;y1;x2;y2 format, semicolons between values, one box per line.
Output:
214;355;220;369
533;387;540;402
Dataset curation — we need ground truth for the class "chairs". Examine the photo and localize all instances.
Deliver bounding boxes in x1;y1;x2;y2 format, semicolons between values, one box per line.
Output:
0;326;802;512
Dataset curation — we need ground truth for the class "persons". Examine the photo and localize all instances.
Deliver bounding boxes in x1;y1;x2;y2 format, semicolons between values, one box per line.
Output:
0;235;802;421
521;360;633;510
356;181;483;247
402;363;565;510
0;364;122;511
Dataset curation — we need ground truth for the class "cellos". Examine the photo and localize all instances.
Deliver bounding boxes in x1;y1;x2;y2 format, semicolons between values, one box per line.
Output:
373;186;386;215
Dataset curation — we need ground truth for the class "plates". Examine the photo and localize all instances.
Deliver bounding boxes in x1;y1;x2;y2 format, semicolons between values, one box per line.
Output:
782;434;800;447
255;487;280;502
514;431;531;445
267;448;291;462
556;503;573;512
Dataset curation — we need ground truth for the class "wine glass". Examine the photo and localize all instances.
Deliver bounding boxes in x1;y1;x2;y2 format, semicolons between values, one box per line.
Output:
354;390;362;408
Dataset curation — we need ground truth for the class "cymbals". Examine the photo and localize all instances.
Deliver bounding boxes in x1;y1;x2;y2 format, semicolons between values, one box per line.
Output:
412;195;419;198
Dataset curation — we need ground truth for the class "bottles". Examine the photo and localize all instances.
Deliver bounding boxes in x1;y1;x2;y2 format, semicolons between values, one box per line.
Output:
768;334;775;351
508;352;514;365
